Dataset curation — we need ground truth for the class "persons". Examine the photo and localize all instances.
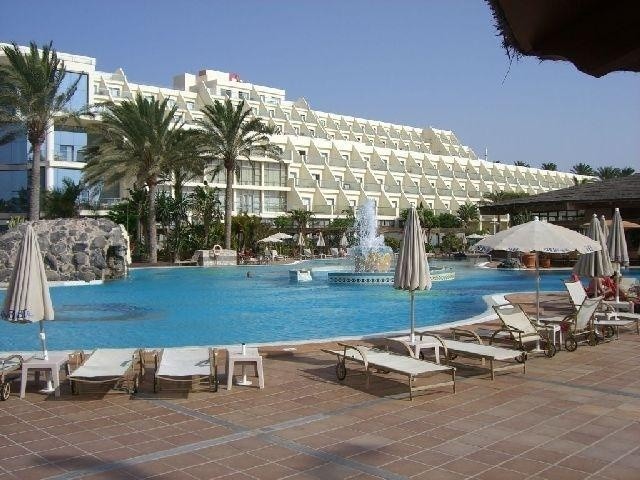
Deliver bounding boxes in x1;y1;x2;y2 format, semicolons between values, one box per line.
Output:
587;277;615;301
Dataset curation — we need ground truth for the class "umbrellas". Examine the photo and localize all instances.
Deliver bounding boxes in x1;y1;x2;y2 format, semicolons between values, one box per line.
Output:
466;233;485;239
422;230;429;251
473;213;602;343
257;236;282;244
270;232;293;256
598;216;608;239
583;219;640;229
338;234;349;247
315;233;325;246
572;215;610;299
393;203;430;346
295;232;305;255
605;209;631;302
0;222;60;388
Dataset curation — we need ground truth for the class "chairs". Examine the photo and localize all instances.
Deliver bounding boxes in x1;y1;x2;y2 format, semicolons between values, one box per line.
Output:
64;348;218;398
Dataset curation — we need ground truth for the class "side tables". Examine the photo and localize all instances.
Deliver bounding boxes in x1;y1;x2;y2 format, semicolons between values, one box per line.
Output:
19;353;72;398
226;348;264;390
